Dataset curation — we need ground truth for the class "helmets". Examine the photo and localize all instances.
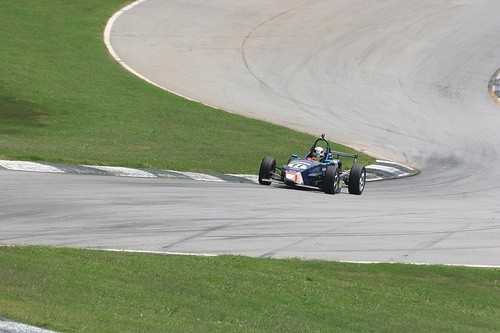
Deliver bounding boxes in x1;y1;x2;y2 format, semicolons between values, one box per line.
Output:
313;146;324;157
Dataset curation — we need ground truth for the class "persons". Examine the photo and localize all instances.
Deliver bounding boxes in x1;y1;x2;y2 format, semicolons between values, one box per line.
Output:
307;146;326;161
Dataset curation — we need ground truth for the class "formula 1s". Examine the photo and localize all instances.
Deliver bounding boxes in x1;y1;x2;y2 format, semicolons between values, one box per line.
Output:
258;133;367;196
